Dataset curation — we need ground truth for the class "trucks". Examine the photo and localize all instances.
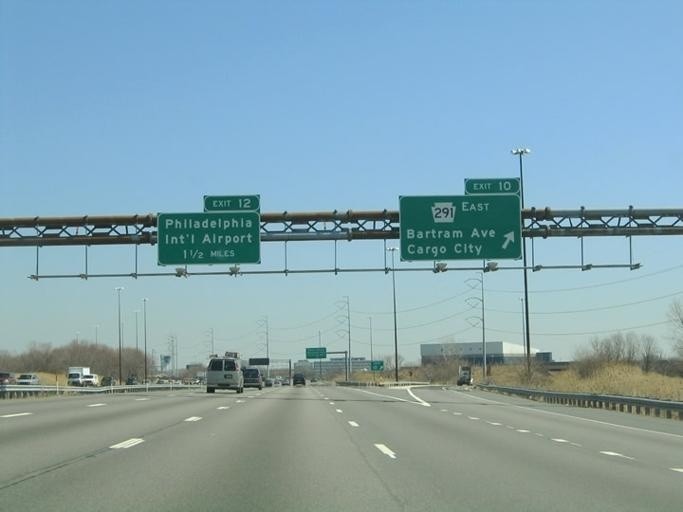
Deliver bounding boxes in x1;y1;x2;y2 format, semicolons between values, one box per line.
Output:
67;367;90;386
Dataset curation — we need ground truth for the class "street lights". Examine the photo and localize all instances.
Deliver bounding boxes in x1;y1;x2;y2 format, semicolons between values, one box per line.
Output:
511;147;531;372
114;287;124;385
366;316;374;371
386;247;399;382
132;298;149;392
95;324;101;345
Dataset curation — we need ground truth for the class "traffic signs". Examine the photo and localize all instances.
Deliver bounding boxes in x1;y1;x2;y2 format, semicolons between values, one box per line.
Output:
398;194;522;259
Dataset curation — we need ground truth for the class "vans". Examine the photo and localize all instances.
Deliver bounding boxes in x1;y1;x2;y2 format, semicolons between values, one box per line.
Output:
457;365;473;386
206;357;244;393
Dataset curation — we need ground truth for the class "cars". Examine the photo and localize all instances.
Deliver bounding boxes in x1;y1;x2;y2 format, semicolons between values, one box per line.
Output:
0;372;40;385
174;376;207;384
243;367;317;390
82;374;151;387
